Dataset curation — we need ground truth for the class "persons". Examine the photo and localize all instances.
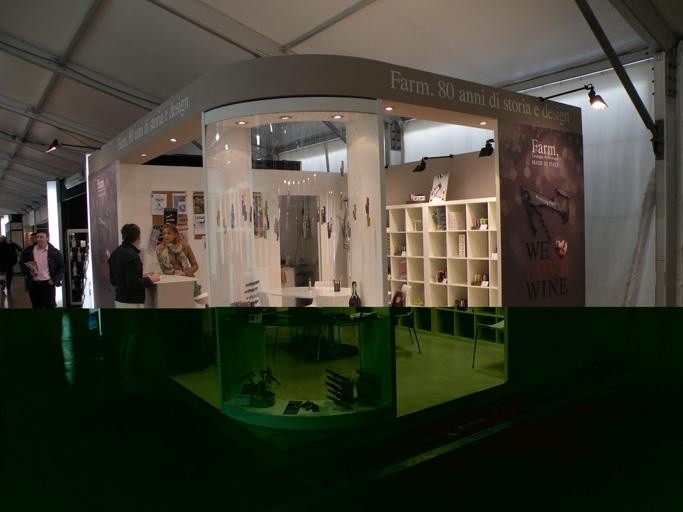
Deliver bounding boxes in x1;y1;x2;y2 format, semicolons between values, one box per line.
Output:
18;229;64;309
0;234;23;293
108;223;160;311
154;224;202;301
29;231;37;242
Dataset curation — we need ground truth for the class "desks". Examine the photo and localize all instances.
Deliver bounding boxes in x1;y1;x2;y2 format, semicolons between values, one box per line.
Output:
267;286;353;361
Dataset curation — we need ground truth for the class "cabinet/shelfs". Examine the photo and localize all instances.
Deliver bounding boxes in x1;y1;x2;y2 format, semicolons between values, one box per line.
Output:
67;229;89;306
144;276;196;307
384;196;506;345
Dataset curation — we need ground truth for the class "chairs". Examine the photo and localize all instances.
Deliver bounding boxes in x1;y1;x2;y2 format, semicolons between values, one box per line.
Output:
313;280;334;286
328;307;376;349
472;300;505;368
390;284;423;355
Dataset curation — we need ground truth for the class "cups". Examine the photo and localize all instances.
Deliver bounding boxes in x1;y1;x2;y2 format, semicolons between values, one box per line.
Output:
334;279;340;291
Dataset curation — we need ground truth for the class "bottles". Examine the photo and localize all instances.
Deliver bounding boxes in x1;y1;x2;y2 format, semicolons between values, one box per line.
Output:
308;278;313;299
349;281;361;307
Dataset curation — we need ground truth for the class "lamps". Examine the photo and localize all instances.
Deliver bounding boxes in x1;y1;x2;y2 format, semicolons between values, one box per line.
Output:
413;153;453;171
538;83;608;112
45;139;100;153
479;139;496;156
